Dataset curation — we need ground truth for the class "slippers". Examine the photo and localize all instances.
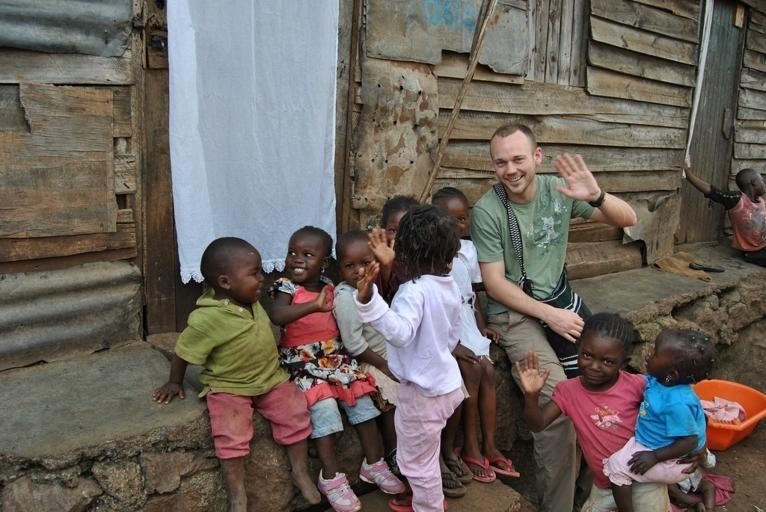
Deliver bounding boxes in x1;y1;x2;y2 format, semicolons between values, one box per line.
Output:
490;457;521;479
453;446;497;484
387;494;448;512
441;471;467;498
444;455;474;484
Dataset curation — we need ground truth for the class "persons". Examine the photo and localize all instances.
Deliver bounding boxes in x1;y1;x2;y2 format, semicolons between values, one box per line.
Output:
331;231;402;475
468;124;636;512
603;326;715;511
269;225;406;512
381;195;472;497
152;237;322;511
352;203;465;512
431;187;521;484
668;411;715;511
683;160;766;267
516;311;704;512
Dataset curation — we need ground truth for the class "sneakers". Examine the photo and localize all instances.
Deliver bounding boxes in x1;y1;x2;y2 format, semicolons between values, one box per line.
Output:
318;468;362;512
358;457;407;494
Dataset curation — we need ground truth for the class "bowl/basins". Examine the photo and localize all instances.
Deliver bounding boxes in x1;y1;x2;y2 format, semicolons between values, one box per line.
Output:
691;379;766;450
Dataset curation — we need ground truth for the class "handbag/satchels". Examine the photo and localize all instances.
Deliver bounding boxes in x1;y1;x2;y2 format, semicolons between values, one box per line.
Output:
493;181;594;379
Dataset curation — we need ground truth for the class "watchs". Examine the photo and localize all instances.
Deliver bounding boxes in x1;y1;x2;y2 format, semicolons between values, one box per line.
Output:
588;188;605;208
